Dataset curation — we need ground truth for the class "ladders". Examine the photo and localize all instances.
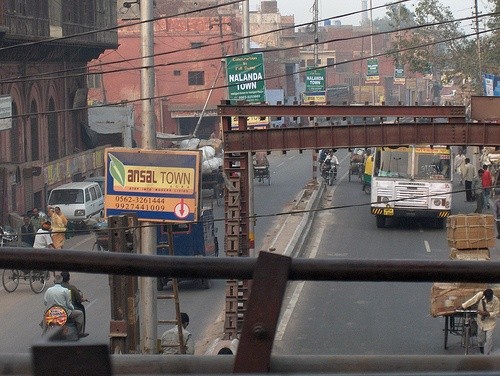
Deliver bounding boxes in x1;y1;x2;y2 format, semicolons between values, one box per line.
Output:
134;221;188;356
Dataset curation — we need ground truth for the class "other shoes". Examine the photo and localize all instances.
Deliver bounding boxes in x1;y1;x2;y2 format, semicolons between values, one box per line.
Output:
465;199;473;202
496;236;500;239
487;204;490;209
78;332;89;339
258;179;261;182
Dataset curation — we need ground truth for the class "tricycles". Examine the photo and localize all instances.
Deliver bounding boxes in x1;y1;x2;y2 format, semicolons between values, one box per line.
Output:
348;162;365;181
253;165;271;186
442;308;479;356
202;167;224;206
2;266;56;294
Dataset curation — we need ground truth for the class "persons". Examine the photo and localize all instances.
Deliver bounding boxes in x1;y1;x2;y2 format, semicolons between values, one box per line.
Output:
461;288;500;356
20;204;68;270
349;147;372;174
42;271;89;339
318;148;341;182
218;347;233;356
454;148;467;186
481;164;493;210
460;158;476;201
160;312;193;356
255;152;270;183
472;169;485;214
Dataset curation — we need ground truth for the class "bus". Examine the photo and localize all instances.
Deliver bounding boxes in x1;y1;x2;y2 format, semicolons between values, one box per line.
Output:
370;147;453;230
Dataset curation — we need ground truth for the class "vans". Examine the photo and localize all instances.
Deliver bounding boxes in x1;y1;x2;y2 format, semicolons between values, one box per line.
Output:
45;181;104;225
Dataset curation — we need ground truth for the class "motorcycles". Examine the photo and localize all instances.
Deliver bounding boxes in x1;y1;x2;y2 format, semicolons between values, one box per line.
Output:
362;155;374;194
322;161;338;186
45;297;89;341
157;205;219;290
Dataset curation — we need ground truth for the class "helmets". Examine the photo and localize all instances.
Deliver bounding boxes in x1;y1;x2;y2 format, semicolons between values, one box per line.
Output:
41;218;52;230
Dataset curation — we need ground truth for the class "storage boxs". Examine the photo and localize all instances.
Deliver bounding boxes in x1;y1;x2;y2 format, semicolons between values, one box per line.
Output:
431;214;500;318
